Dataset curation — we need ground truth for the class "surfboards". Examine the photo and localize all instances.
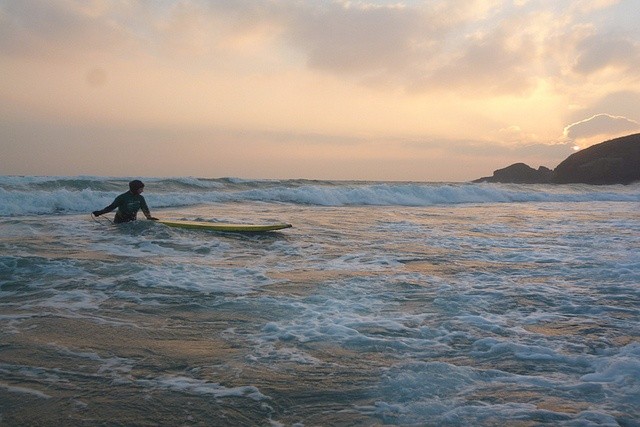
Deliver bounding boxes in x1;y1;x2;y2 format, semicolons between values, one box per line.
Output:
139;218;292;233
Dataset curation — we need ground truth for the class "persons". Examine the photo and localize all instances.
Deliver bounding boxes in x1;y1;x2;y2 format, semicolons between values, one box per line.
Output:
93;179;159;223
